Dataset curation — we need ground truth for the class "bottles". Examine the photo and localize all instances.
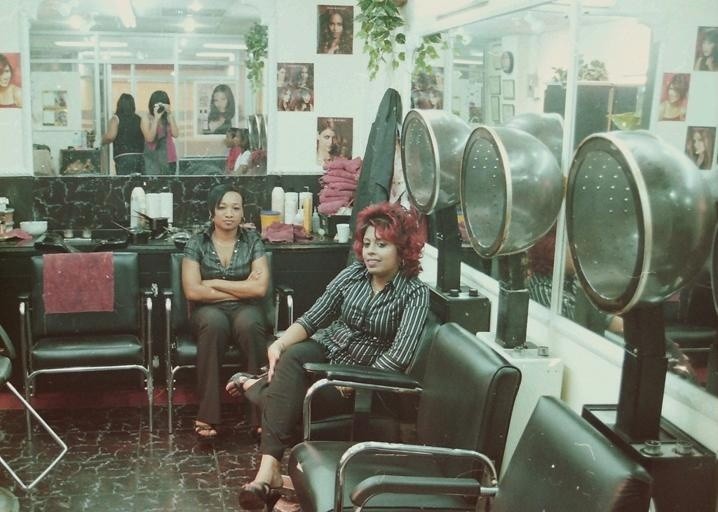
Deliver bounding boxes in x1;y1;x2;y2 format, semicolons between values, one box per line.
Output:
128;182;173;231
198;109;209;130
260;179;322;236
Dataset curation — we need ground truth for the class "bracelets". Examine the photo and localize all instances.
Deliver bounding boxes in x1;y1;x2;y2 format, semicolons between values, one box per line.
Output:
338;385;354;399
274;340;284;352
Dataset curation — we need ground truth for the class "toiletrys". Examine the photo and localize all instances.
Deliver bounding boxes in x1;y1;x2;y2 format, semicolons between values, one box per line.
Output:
302;196;312;233
271;181;285;227
130;181;144;231
284;186;299;223
160;186;174;224
299;185;313;229
145;187;160;218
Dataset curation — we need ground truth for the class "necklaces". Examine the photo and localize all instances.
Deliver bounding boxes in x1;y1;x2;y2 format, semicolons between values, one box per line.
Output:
371;279;384;294
214;231;237;246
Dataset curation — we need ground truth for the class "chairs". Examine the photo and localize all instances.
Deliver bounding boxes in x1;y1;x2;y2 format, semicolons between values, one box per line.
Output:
15;253;158;441
161;251;297;434
0;324;69;493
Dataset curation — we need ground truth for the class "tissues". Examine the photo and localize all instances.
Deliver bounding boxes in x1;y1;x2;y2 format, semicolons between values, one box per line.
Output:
0;195;15;225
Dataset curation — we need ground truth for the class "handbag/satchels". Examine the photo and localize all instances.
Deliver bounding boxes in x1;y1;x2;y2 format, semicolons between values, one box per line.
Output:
143;145;170;175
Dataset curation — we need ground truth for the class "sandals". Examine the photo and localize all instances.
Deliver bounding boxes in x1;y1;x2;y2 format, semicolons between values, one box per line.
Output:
193;418;219;441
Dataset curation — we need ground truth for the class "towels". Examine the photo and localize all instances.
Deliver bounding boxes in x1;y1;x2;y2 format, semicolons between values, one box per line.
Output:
258;221;314;245
245;148;268;177
315;154;363;218
42;252;114;314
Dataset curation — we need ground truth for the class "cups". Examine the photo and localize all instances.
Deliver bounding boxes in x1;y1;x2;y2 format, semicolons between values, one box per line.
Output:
336;224;350;243
125;216;173;245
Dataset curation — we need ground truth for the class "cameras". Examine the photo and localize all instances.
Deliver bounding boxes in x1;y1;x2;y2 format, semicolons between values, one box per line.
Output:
158;104;169;113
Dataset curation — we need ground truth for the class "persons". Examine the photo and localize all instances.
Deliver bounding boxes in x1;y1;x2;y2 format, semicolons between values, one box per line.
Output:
523;224;695;382
317;119;342;165
695;29;717;71
319;8;353;54
686;127;712;169
182;183;269;443
140;91;178;175
204;86;235;134
100;94;145;175
225;127;267;175
0;54;23;107
658;73;689;121
277;65;314;111
224;202;429;512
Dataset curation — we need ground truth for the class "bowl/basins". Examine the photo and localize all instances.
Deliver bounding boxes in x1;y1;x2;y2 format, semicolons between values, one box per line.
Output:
173;238;189;249
19;220;48;236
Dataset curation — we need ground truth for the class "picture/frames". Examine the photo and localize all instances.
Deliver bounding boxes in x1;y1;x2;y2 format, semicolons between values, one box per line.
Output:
490;96;500;122
502;104;515;122
488;75;501;95
502;79;515;99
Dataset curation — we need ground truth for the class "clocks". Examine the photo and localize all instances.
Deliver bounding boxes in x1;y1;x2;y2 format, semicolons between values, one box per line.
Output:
500;51;513;74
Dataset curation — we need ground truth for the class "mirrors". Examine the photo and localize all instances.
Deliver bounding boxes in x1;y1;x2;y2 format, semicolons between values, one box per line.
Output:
406;0;718;420
0;0;411;178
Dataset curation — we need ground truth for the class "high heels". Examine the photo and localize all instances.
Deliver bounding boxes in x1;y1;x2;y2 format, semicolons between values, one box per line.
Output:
225;364;275;399
237;480;284;510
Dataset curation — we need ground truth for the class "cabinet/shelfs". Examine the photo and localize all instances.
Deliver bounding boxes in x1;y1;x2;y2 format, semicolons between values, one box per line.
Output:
59;146;101;177
263;235;353;331
